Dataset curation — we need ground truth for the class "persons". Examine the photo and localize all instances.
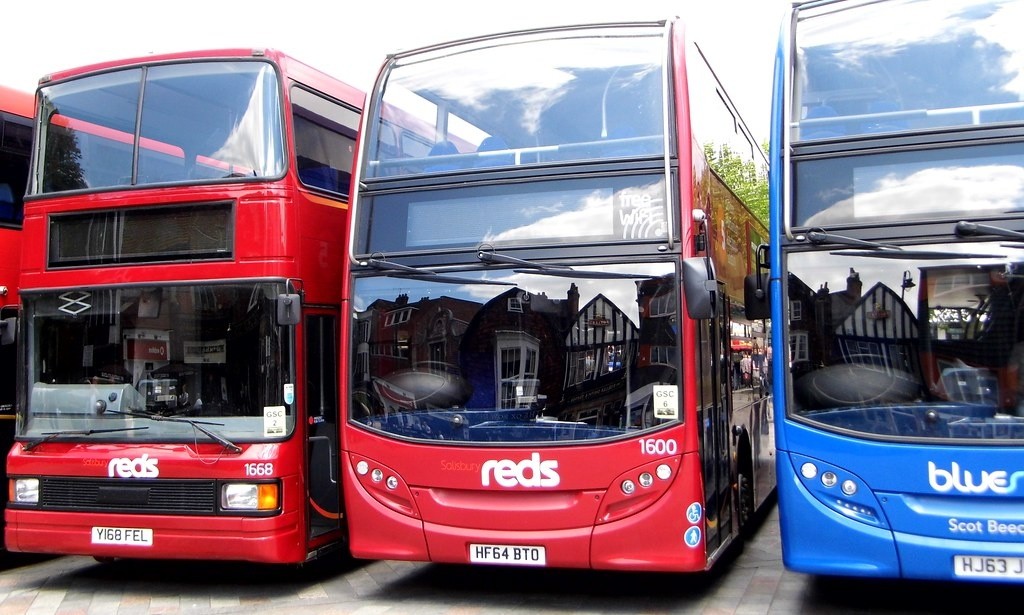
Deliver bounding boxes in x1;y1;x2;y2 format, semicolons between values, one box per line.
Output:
178;384;202;410
730;355;758;390
608;346;622;362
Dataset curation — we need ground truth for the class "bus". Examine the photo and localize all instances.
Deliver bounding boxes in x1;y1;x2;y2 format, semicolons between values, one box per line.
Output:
339;21;777;575
743;1;1024;589
2;46;475;580
0;81;262;569
